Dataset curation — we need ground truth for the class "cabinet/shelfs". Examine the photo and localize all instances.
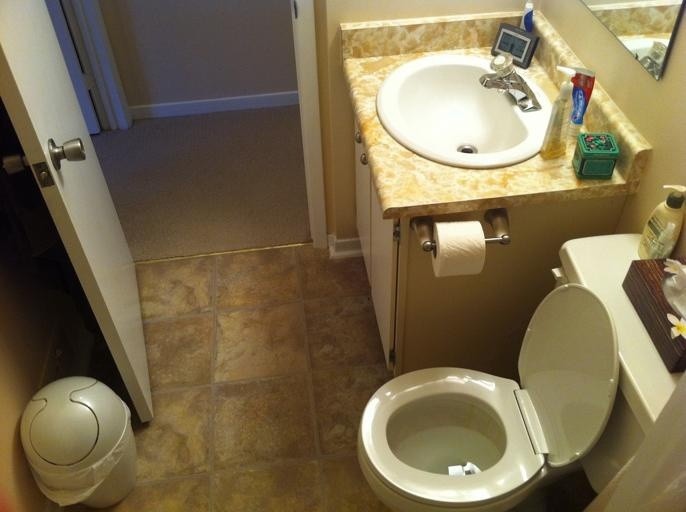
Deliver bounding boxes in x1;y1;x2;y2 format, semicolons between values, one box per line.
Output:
355;109;627;379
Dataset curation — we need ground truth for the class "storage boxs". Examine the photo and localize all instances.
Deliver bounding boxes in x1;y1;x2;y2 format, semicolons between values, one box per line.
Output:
622;258;682;374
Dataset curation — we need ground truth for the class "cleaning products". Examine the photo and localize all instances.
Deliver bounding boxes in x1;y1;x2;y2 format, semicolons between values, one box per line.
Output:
648;223;676;259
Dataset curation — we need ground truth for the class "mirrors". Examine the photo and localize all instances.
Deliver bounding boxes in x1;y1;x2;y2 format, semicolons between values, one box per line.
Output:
578;0;686;84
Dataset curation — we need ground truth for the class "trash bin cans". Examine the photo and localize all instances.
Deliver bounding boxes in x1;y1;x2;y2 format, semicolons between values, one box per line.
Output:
20;376;137;509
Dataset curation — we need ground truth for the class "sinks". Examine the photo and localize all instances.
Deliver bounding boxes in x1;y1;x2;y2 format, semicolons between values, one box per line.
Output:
617;37;669;61
377;54;552;169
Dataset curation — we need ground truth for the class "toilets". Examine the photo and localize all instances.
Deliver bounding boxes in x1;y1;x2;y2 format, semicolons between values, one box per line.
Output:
357;283;618;510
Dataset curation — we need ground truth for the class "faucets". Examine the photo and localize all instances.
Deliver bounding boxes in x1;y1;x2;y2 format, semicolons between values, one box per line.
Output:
479;73;510;93
